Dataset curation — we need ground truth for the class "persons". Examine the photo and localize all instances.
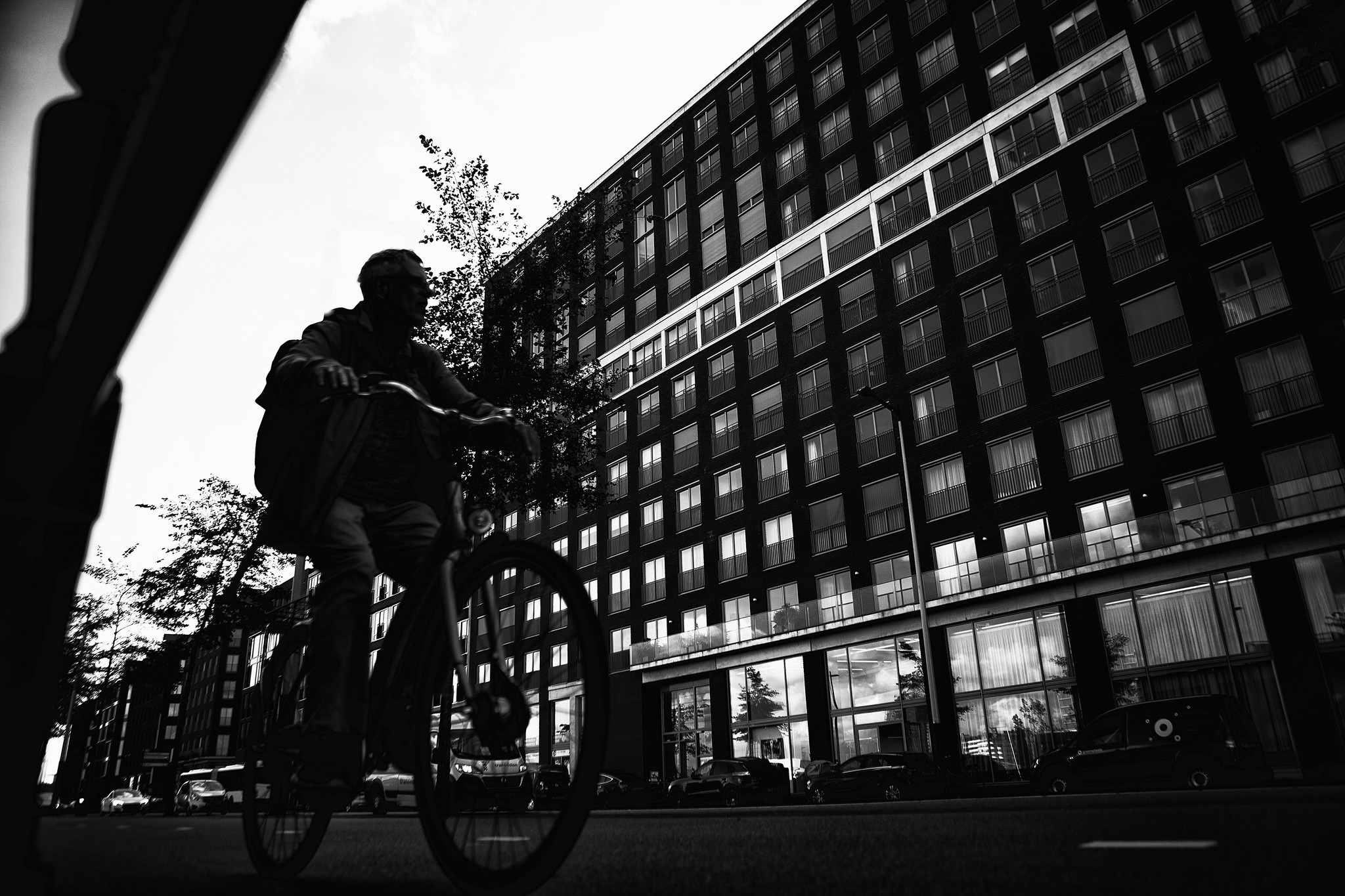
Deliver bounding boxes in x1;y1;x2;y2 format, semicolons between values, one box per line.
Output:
254;249;543;814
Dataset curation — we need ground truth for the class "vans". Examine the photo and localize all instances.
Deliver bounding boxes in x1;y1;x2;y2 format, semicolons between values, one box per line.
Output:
1030;691;1275;796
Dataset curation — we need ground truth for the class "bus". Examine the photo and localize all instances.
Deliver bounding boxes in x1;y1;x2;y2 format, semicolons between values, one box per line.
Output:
178;760;271;809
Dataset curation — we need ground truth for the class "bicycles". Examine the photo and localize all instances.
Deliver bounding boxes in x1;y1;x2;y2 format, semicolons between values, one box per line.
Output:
239;375;612;896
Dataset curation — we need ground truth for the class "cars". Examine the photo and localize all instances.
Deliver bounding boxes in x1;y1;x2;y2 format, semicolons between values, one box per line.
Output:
35;791;62;811
100;789;151;815
808;752;941;803
666;755;791;806
533;771;644;808
173;779;230;815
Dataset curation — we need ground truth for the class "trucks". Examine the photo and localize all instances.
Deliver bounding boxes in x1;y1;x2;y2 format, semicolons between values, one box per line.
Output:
362;714;531;815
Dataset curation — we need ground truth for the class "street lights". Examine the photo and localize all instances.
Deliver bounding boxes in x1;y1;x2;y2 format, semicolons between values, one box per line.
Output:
855;384;943;763
